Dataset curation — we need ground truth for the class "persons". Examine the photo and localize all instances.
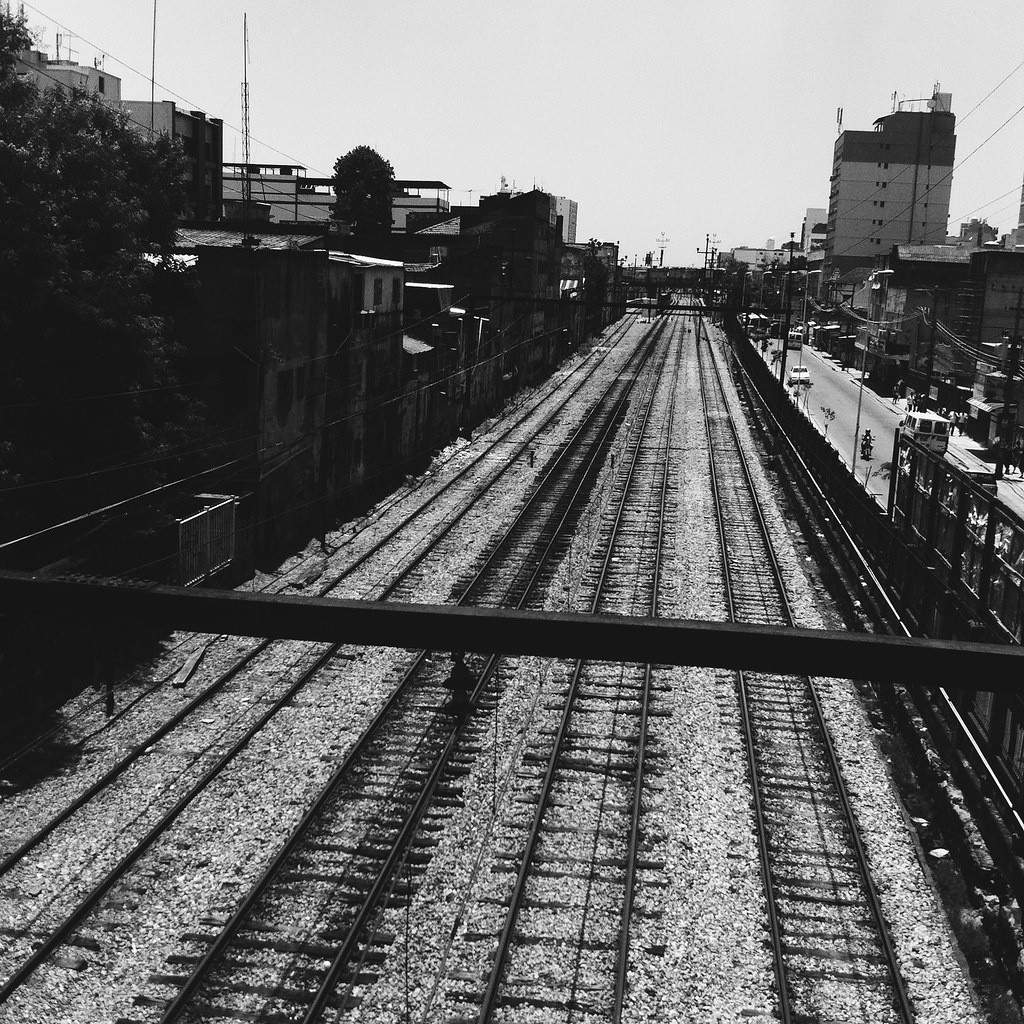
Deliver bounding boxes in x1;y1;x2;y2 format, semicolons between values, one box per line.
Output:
908;392;920;412
947;409;967;436
861;429;873;451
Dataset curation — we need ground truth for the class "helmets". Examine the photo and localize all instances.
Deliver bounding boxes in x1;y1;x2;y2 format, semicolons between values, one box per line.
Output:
865;429;871;433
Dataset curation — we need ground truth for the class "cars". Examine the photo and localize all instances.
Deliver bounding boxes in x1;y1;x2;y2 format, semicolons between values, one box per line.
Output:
789;366;809;385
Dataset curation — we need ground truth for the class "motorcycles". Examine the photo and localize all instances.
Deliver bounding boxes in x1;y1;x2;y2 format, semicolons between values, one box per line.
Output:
859;434;876;461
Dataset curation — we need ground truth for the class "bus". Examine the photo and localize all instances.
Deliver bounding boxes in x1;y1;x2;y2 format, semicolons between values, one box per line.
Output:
901;412;951;455
789;330;803;352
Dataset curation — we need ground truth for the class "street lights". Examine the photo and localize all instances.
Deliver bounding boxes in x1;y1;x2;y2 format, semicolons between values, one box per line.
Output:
654;231;672;265
731;268;773;352
780;241;805;386
851;268;896;473
783;269;800;382
710;233;719;301
796;269;824;400
